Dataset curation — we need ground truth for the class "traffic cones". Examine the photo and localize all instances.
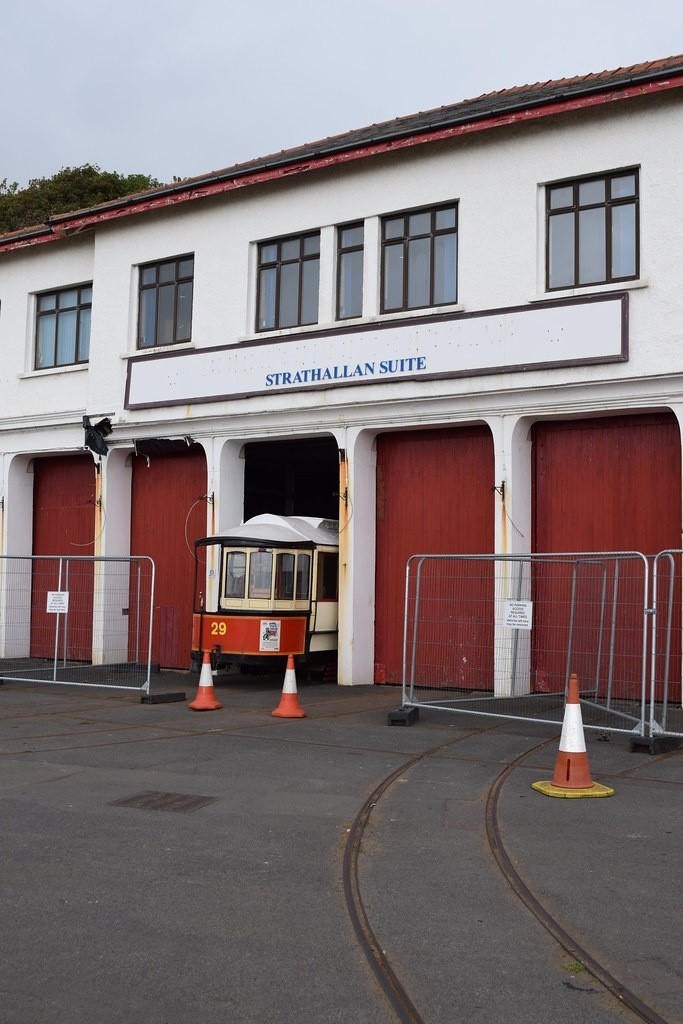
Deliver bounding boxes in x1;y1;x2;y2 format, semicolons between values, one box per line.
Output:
271;653;308;719
528;671;617;799
185;649;224;710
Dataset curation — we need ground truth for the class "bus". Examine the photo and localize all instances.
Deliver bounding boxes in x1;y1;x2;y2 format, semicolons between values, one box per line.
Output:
188;514;339;677
